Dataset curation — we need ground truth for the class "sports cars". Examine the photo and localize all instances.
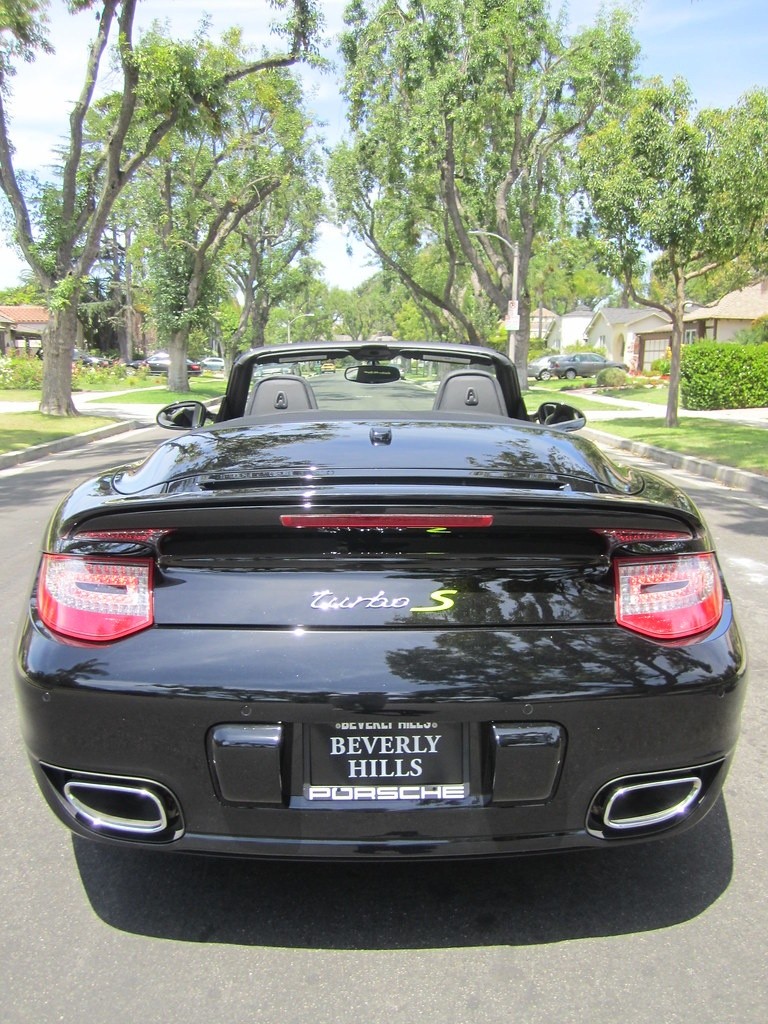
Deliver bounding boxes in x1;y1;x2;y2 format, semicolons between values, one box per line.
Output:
13;340;747;863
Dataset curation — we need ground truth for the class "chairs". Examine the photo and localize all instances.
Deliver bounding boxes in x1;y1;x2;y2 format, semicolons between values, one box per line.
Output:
243;375;318;417
432;369;508;417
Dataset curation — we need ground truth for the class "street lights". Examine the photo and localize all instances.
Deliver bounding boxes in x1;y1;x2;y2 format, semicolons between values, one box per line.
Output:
466;230;520;365
287;314;314;344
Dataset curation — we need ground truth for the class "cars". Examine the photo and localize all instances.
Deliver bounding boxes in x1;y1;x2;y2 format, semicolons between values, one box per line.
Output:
525;355;568;381
418;361;429;367
253;362;301;384
320;364;336;373
547;352;630;380
125;353;203;379
390;364;407;380
201;358;224;370
36;347;114;374
